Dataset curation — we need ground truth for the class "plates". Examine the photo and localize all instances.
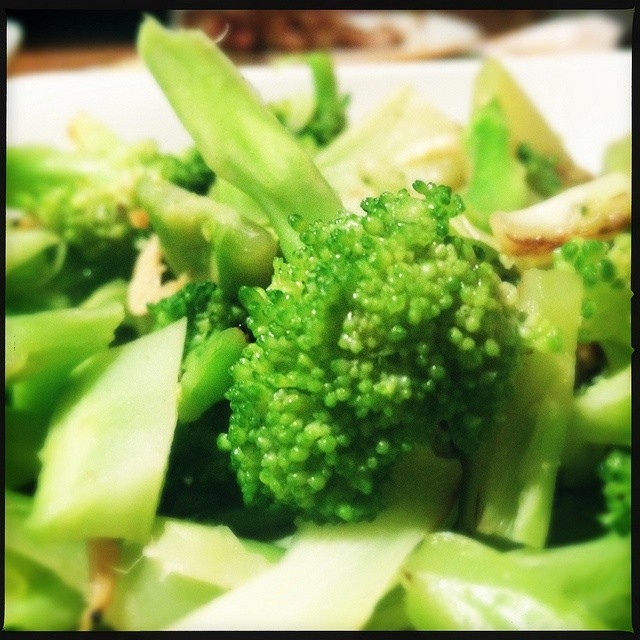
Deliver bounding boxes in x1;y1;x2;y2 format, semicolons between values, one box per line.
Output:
5;56;631;633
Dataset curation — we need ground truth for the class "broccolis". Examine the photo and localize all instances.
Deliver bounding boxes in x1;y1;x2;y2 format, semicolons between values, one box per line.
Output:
2;17;636;628
